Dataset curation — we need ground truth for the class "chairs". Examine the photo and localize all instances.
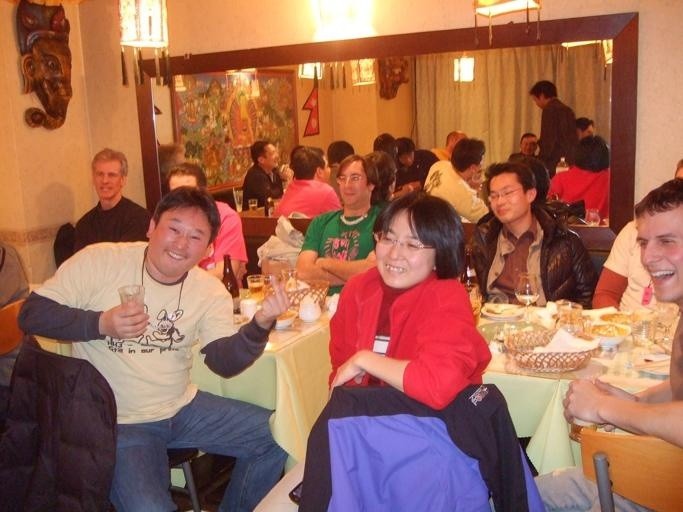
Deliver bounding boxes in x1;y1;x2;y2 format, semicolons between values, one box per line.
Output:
5;341;205;510
0;298;29;399
50;221;76;273
576;419;683;511
282;382;545;512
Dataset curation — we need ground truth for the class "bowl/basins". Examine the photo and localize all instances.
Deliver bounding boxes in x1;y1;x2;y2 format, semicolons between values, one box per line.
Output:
274;309;298;330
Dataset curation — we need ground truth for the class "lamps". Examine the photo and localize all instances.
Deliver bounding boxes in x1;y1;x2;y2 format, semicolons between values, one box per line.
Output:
470;0;547;50
347;56;377;90
114;1;172;91
452;50;475;86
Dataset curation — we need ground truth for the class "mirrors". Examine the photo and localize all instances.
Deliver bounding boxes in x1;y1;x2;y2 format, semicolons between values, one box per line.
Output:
127;7;641;266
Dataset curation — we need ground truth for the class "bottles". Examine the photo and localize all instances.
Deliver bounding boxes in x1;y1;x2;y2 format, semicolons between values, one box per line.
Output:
219;253;240;314
463;246;478;286
263;184;272;217
555;157;570;177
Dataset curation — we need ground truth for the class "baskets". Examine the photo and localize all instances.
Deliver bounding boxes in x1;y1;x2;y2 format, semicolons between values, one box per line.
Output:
503;329;598;373
269;280;330;318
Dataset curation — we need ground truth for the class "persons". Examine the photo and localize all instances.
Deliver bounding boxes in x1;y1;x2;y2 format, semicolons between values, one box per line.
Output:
326;190;493;409
489;177;683;511
295;154;393;296
462;161;600;308
529;80;577;178
69;148;153;252
546;118;611;226
167;161;247;289
16;184;297;512
276;132;541;226
0;238;33;416
242;141;294;213
591;158;683;310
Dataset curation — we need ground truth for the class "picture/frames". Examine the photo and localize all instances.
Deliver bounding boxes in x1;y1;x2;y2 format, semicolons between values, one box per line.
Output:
165;69;302;199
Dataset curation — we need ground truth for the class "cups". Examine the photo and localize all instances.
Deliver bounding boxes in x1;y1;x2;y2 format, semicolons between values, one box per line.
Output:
240;264;322;322
246;199;258;212
229;182;244;214
582;208;602;228
471;169;486;186
117;285;144;306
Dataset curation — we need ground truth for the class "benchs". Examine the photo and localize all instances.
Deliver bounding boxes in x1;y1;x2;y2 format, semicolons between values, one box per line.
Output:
229;211;625;310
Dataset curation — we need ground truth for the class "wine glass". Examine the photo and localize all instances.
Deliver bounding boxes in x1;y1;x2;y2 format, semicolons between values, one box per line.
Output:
466;273;678;373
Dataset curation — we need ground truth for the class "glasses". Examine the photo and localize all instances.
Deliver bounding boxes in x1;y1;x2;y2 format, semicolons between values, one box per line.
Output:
488;188;521;203
372;231;435;251
335;175;362;184
